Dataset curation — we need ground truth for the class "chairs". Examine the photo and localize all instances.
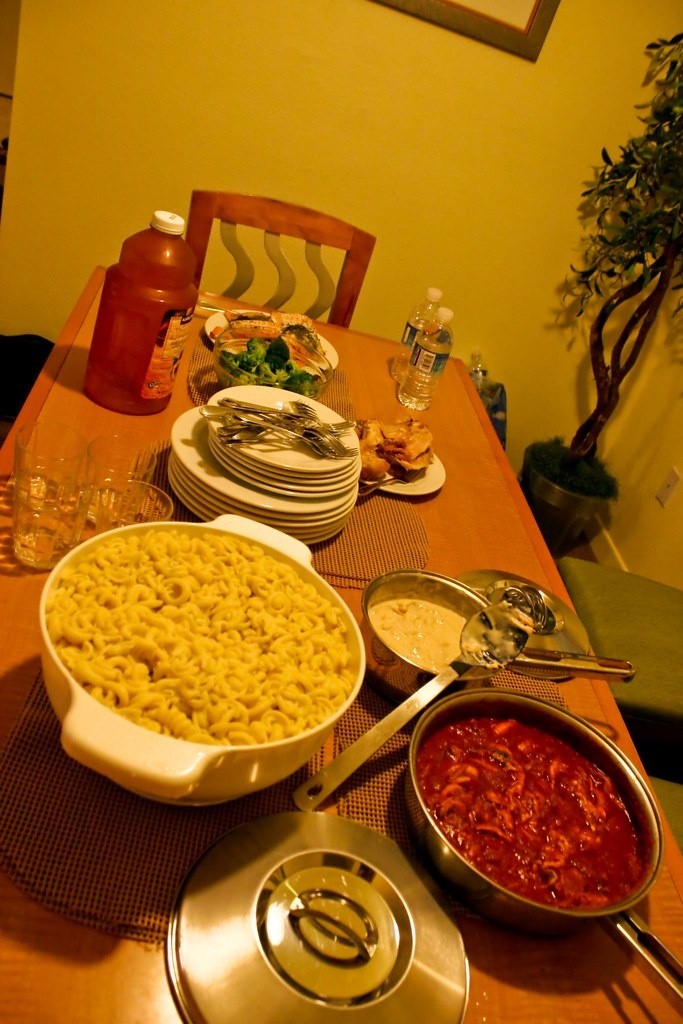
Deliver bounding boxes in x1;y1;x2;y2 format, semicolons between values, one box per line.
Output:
178;188;377;328
557;557;683;862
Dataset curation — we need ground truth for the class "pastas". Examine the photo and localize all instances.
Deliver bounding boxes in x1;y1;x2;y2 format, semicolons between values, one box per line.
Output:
45;529;356;746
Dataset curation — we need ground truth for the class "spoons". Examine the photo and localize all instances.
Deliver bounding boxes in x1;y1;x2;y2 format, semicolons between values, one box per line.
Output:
293;602;535;813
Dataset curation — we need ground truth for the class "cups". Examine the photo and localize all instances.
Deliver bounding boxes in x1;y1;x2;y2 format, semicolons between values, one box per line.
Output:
12;421;94;571
73;437;155;549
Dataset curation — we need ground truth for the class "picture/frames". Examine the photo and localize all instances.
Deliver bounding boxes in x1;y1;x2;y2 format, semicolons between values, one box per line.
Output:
365;0;561;64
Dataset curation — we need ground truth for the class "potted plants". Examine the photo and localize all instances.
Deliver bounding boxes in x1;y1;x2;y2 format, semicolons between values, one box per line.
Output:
515;34;683;562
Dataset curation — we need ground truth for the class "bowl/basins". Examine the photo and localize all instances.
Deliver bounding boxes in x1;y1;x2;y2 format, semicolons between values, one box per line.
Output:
37;513;367;807
212;328;335;401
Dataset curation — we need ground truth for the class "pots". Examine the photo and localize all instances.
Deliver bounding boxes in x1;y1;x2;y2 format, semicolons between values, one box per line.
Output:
404;688;683;1001
357;570;638;715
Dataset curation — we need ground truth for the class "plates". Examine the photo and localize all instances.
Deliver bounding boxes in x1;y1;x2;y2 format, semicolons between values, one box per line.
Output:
359;449;446;496
89;479;174;539
205;309;339;370
166;385;362;546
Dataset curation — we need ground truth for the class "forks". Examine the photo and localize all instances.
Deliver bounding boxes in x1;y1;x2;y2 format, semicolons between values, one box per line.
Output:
355;468;424;496
198;399;359;458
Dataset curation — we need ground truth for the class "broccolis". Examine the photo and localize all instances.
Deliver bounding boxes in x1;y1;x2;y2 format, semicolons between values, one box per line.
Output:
219;339;315;396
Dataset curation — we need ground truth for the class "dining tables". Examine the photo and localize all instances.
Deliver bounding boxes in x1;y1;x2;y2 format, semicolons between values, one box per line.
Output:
0;264;683;1024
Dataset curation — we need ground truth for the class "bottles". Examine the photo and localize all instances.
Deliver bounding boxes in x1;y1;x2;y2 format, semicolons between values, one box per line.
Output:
468;351;487;394
390;286;444;384
397;308;457;410
83;210;198;415
